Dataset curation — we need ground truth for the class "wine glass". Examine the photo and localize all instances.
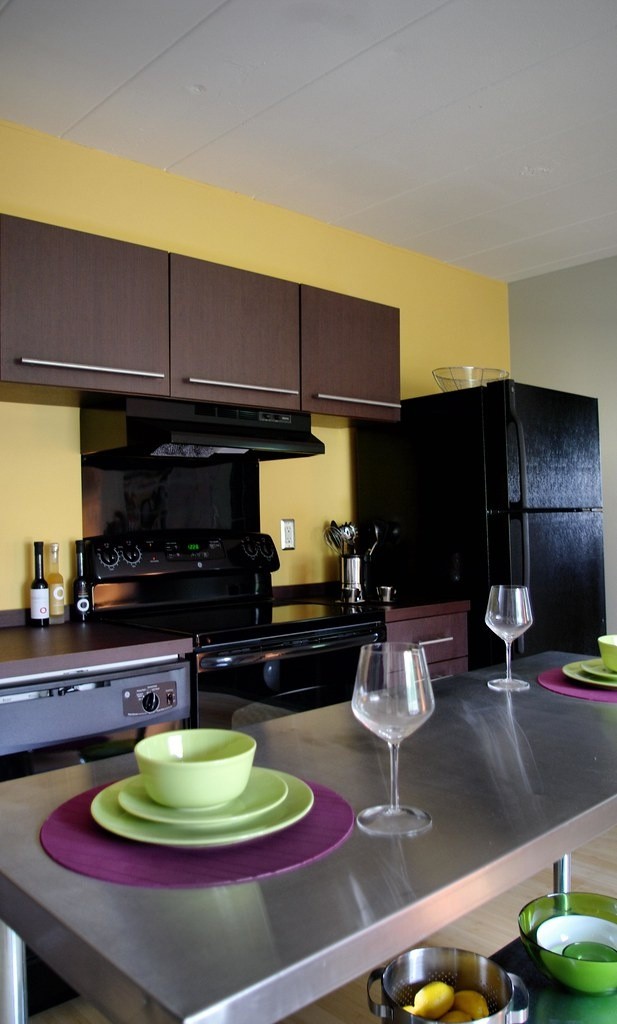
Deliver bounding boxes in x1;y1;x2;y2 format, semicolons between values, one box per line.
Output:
485;585;533;691
352;642;435;838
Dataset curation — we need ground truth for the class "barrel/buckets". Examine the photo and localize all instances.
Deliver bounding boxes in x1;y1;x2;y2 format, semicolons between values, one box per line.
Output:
338;554;370;601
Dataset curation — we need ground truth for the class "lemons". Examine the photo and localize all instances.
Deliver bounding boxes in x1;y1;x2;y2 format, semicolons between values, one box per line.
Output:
401;981;489;1023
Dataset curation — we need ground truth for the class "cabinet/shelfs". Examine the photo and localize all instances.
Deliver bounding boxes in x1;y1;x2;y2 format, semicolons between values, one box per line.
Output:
385;603;467;686
0;212;401;421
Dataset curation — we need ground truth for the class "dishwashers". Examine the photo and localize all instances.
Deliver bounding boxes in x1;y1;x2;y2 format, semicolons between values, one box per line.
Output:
0;661;192;1024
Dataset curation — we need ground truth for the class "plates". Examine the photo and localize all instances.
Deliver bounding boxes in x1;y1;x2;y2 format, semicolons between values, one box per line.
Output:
91;767;315;849
562;660;617;690
118;767;288;831
581;659;617;681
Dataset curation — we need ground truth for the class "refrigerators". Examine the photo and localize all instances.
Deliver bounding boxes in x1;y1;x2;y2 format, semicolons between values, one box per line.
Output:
348;378;607;671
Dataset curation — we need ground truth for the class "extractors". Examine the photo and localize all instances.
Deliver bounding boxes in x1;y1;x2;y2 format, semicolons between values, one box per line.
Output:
79;392;325;460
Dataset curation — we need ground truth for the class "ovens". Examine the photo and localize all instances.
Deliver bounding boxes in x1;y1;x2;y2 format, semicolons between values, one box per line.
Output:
185;624;383;727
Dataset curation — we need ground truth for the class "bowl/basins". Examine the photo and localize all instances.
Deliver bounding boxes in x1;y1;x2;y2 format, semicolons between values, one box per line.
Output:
432;366;510;393
519;893;617;996
597;634;617;673
134;728;257;813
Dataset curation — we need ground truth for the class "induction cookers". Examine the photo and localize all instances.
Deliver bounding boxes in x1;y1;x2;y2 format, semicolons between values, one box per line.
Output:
83;530;386;647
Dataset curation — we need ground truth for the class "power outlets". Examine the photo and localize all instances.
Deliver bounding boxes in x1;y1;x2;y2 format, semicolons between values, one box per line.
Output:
281;518;295;550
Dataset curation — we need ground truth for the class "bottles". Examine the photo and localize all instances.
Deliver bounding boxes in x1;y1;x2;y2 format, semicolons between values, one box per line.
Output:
30;542;48;627
47;543;65;625
72;540;92;623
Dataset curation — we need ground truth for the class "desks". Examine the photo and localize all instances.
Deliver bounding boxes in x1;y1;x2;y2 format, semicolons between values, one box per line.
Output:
0;651;617;1024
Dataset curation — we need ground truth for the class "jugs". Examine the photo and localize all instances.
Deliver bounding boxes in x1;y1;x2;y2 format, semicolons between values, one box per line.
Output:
376;586;396;602
340;586;361;603
